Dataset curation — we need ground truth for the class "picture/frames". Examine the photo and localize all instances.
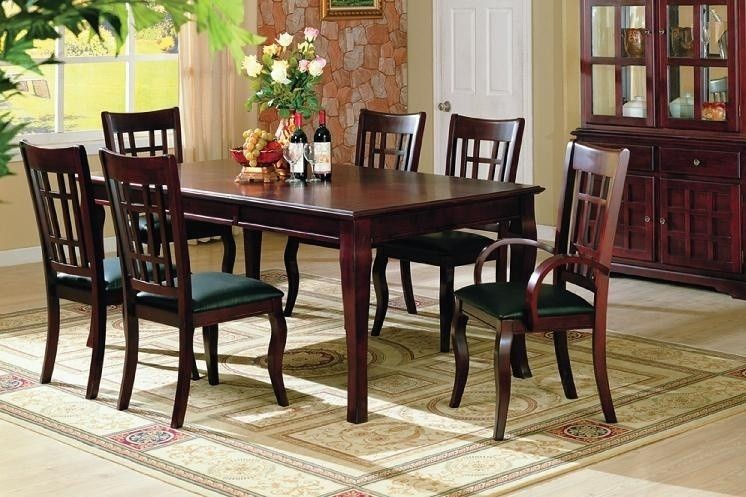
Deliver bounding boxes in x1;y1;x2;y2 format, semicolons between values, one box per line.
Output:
317;0;385;21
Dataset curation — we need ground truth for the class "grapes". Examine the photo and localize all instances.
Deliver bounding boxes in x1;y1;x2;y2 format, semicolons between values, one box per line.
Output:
241;128;273;166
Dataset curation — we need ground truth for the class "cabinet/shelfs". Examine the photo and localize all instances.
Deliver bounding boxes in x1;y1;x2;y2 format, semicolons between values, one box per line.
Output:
572;1;746;133
568;125;746;303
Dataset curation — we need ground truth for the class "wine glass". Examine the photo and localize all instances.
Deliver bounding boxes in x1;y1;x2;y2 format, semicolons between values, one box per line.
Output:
703;22;711;58
282;144;303;183
304;144;320;183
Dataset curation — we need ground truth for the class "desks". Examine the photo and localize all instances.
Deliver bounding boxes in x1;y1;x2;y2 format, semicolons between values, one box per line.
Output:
71;153;546;427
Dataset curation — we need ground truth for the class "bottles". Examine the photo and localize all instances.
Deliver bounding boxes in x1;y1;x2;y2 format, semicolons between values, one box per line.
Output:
291;115;308;180
312;110;332;180
710;7;725;23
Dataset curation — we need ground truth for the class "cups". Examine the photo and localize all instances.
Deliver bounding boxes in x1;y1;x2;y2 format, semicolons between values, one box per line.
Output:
671;25;692;57
622;27;645;58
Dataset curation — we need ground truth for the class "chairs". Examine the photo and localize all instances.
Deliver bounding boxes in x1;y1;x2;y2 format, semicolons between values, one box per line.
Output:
98;106;238;279
96;147;290;429
282;107;428;320
442;138;630;443
16;138;201;405
369;111;525;354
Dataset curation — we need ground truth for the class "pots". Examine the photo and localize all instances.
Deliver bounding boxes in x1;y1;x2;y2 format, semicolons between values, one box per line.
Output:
668;93;694;118
622;95;648;119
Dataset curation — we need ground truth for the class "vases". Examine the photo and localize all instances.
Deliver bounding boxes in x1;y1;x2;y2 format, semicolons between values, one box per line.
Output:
273;116;293;181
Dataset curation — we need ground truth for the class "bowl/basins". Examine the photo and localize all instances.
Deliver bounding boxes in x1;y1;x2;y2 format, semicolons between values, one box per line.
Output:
228;147;283;167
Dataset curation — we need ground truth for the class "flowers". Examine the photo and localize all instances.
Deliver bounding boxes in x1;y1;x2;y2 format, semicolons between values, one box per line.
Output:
232;24;330;122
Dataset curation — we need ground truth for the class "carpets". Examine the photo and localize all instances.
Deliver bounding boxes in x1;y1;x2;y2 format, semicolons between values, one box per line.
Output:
0;267;746;497
